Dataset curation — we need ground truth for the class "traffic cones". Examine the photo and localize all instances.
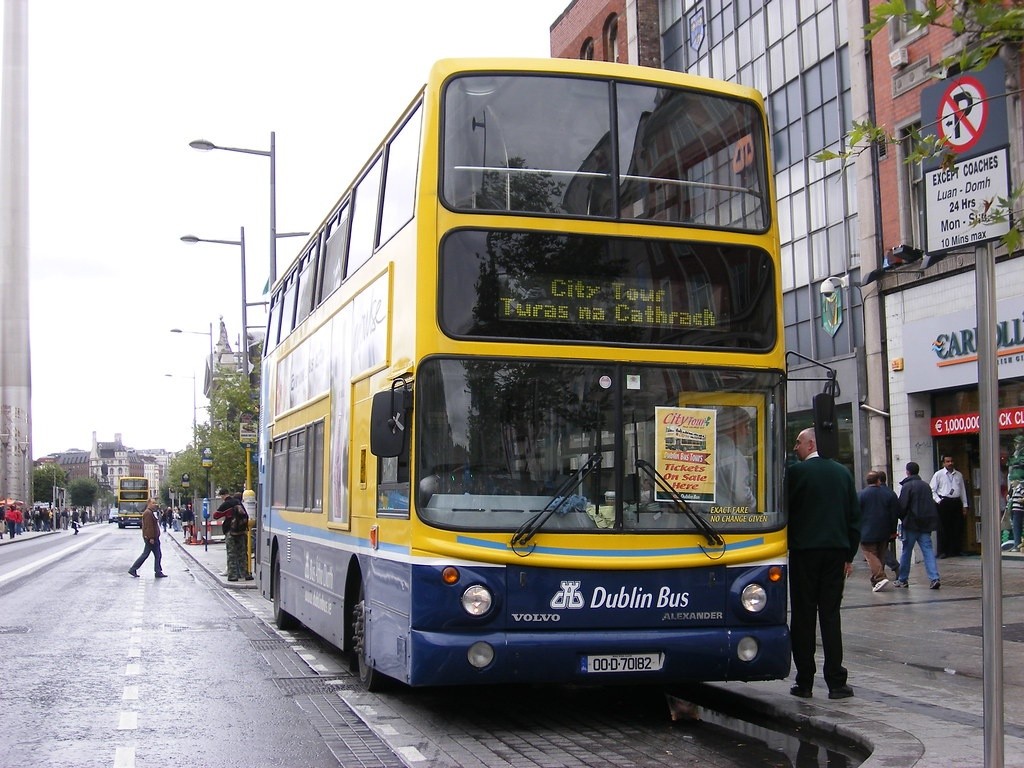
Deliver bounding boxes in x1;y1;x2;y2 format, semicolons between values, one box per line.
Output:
184;524;213;545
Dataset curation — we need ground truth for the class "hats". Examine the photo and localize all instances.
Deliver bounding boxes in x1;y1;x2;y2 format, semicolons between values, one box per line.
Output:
1013;436;1024;444
1009;474;1022;482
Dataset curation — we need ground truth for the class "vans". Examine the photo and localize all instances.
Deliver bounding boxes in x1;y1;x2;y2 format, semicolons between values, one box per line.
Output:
109;508;119;523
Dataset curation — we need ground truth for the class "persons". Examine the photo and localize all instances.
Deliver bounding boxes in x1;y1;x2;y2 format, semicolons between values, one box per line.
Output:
213;488;254;581
928;454;968;559
786;428;860;698
128;498;168;578
0;497;196;539
1009;477;1024;552
893;462;941;590
709;406;756;512
1008;434;1024;482
878;471;901;582
859;471;889;592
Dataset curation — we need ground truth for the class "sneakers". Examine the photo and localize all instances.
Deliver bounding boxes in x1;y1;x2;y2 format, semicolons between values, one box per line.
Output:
929;579;941;589
873;578;889;592
893;580;908;588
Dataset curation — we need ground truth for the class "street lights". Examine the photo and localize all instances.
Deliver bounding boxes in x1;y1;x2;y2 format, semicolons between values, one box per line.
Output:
190;140;277;288
181;235;247;390
166;374;197;532
171;330;215;510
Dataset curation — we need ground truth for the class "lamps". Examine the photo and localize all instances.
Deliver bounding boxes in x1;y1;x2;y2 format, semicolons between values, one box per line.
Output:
859;269;924;287
919;251;975;269
892;245;924;262
820;275;850;293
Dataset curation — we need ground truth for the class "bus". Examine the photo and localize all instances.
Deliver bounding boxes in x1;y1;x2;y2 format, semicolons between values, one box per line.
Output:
119;477;150;530
253;55;843;691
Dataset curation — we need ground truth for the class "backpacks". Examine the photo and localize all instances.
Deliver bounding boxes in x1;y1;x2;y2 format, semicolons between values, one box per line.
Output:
230;504;248;535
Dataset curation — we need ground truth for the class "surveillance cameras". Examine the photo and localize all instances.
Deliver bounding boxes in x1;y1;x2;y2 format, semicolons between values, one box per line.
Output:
820;280;835;298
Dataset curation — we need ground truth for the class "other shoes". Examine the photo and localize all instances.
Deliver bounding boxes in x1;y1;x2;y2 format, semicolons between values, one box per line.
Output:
155;572;167;578
219;572;228;576
939;553;946;559
245;576;254;581
789;683;813;698
128;570;140;577
959;551;971;556
73;530;79;535
828;684;854;700
1009;547;1020;552
